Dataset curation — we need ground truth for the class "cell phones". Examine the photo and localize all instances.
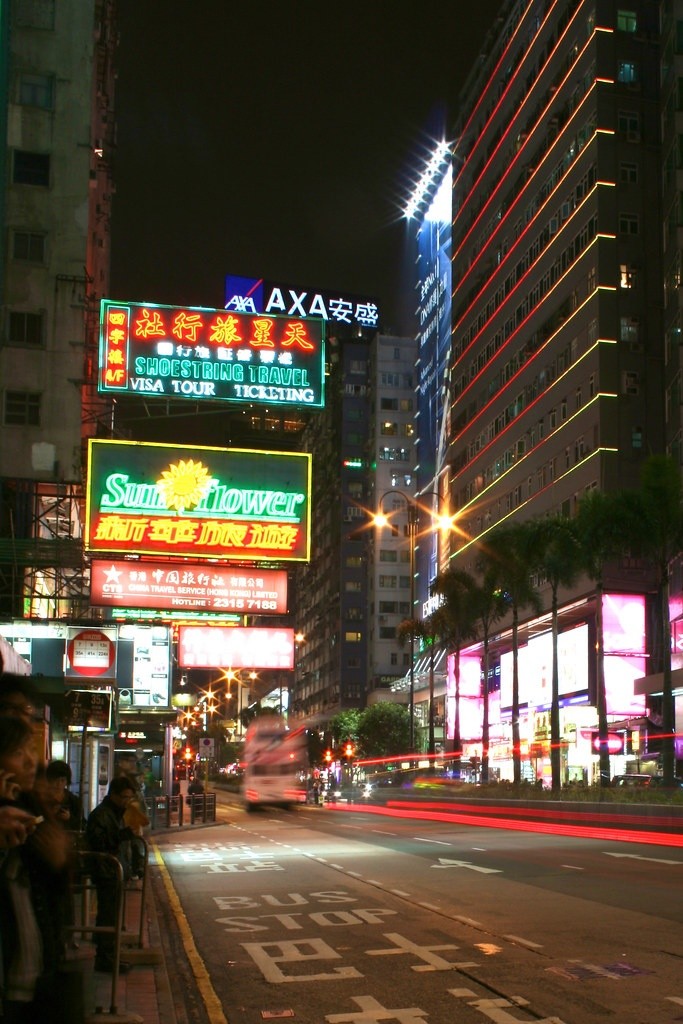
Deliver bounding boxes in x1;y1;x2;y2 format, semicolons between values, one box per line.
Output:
57;806;68;818
22;816;43;826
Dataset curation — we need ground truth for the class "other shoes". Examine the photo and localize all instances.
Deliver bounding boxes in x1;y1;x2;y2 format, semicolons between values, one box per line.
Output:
95;956;130;972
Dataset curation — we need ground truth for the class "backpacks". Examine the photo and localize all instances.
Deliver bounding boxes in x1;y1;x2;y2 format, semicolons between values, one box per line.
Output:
76;834;97;875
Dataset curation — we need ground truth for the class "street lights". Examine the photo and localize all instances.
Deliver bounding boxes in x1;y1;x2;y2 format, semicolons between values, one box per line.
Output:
223;667;260;739
373;490;454;765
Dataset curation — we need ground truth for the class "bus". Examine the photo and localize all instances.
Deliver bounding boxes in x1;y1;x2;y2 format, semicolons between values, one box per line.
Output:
240;728;307;811
368;766;449;797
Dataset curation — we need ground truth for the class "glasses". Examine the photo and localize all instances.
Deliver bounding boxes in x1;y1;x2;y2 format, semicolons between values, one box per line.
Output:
119;794;135;801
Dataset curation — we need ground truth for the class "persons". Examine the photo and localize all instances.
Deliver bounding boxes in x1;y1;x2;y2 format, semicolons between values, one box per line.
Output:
88;777;133;973
0;716;82;1024
187;780;204;802
312;780;324;807
